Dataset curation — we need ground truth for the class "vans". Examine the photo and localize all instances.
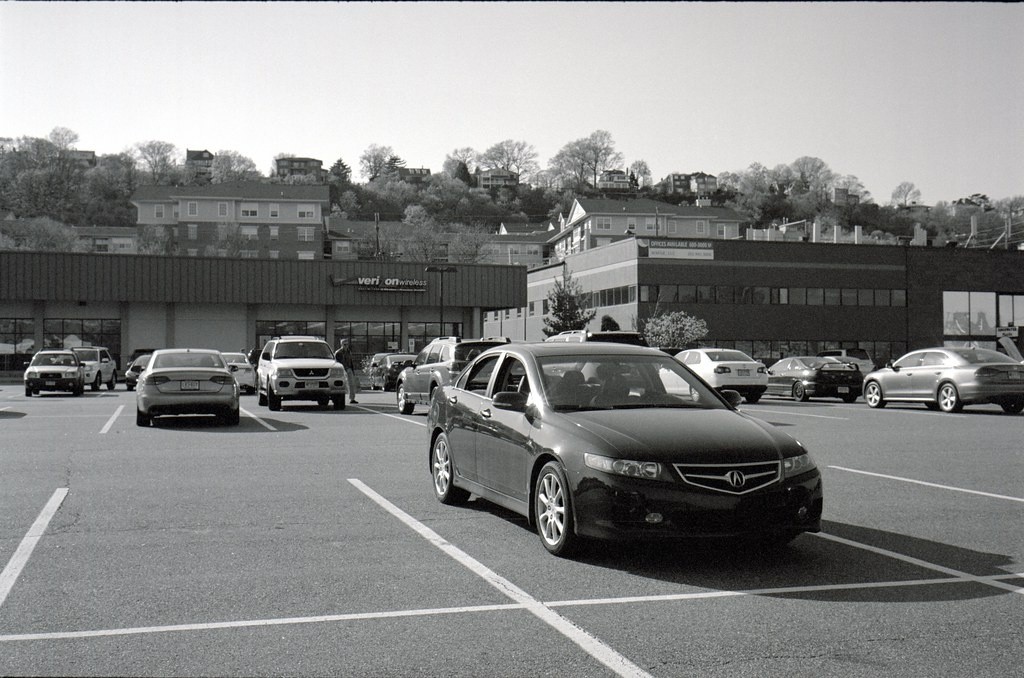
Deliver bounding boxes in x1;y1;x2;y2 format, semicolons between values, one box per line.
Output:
816;347;877;376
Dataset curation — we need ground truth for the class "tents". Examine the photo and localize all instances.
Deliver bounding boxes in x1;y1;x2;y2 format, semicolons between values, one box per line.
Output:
16;334;92;355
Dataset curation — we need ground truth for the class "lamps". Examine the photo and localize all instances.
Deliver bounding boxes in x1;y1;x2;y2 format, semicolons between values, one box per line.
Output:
801;236;810;243
944;239;958;248
624;230;635;236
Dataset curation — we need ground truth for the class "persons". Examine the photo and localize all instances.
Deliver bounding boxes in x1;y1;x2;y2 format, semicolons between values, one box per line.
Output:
334;339;360;404
245;349;259;394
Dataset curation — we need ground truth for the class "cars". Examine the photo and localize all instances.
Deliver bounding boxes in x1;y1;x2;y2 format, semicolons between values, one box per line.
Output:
130;348;241;427
425;341;824;557
366;351;418;391
124;354;152;391
23;347;86;396
763;355;863;403
215;351;257;395
658;348;770;403
862;346;1024;414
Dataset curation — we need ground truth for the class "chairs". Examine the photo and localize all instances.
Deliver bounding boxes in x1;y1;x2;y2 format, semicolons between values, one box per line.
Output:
42;357;52;364
200;355;215;367
561;371;590;404
517;374;558;402
161;356;176;366
596;372;633;406
63;359;72;365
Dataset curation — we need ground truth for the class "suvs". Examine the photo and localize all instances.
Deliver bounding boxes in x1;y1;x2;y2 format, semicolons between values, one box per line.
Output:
396;335;515;415
245;335;349;411
50;345;118;391
538;327;651;390
